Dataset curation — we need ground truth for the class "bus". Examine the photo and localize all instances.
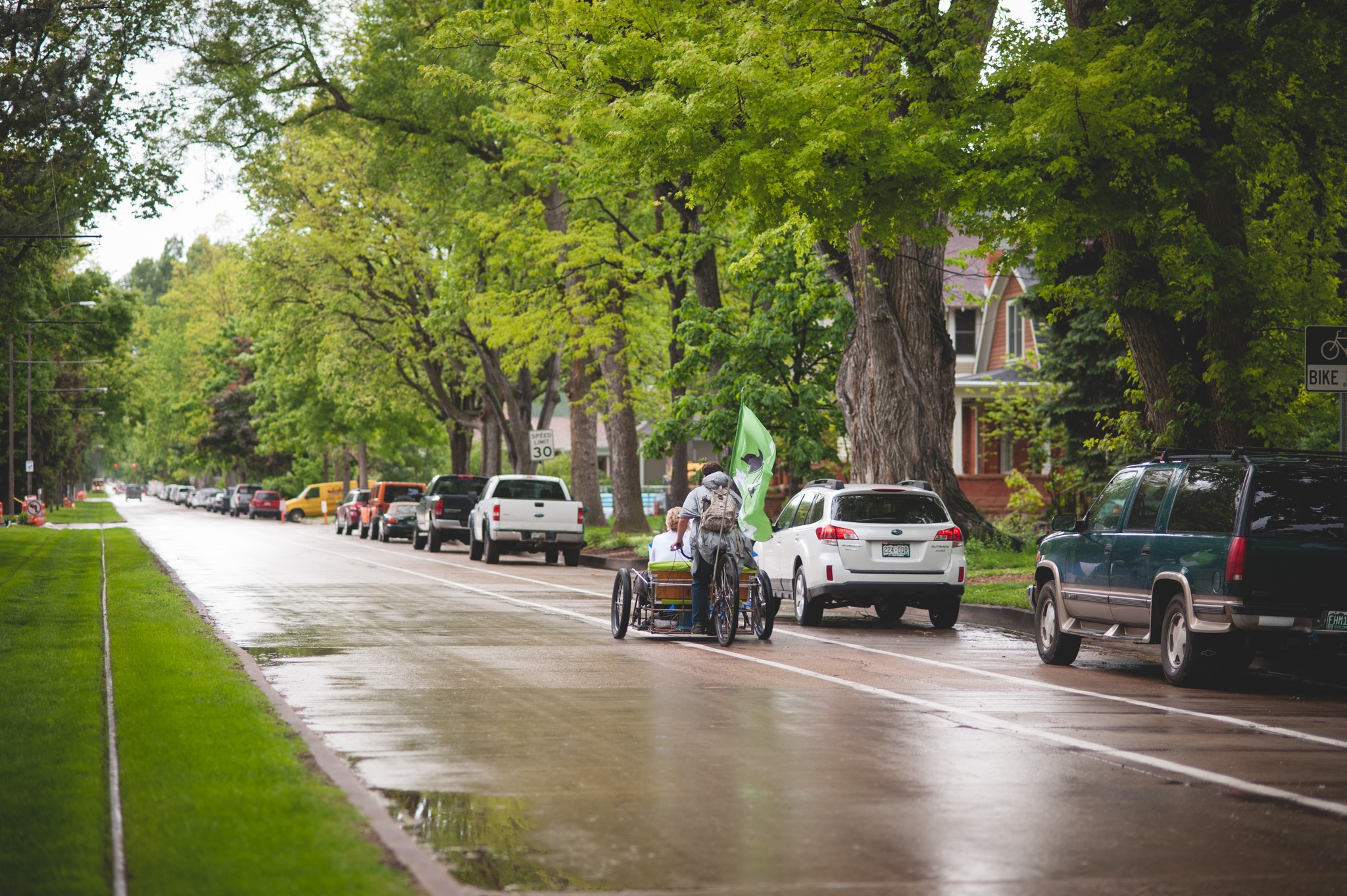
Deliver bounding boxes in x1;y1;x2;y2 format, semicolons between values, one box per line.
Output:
91;478;105;493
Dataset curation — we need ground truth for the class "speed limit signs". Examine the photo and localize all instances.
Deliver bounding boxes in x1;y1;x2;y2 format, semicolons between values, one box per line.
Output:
528;429;554;461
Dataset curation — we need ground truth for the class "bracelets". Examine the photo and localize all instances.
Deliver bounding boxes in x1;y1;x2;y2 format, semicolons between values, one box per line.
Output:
676;539;683;543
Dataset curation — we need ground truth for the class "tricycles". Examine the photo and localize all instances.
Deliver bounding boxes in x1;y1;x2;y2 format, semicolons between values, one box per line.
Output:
610;543;775;647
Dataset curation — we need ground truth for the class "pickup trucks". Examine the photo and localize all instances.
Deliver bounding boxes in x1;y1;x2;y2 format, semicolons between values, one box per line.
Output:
409;473;509;555
467;474;588;567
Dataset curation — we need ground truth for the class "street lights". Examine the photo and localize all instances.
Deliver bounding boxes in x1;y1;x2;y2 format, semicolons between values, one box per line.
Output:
27;300;98;495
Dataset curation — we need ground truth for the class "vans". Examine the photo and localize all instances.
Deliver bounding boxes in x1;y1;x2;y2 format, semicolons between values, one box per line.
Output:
283;479;377;523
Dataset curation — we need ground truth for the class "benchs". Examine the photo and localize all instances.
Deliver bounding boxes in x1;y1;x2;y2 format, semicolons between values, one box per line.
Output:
648;561;757;604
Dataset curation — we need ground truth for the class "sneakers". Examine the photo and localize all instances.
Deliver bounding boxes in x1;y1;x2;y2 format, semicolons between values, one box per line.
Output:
690;622;705;634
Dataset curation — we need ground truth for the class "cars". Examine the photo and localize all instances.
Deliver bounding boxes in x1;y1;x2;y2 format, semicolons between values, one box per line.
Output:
378;501;419;543
247;490;282;521
113;480;236;515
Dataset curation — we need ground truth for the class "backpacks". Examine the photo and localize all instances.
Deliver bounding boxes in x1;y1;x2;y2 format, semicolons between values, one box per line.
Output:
700;485;737;533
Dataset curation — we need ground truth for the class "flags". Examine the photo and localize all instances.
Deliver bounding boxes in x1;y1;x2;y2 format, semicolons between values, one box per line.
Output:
732;401;776;541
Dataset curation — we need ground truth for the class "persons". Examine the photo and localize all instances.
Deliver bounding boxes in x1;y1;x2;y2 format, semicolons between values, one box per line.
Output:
669;462;759;636
650;506;692;562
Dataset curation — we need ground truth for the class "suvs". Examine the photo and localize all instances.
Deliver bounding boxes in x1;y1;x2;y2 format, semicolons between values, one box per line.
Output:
358;481;426;540
226;483;266;518
749;477;968;627
1025;441;1347;688
334;488;371;535
